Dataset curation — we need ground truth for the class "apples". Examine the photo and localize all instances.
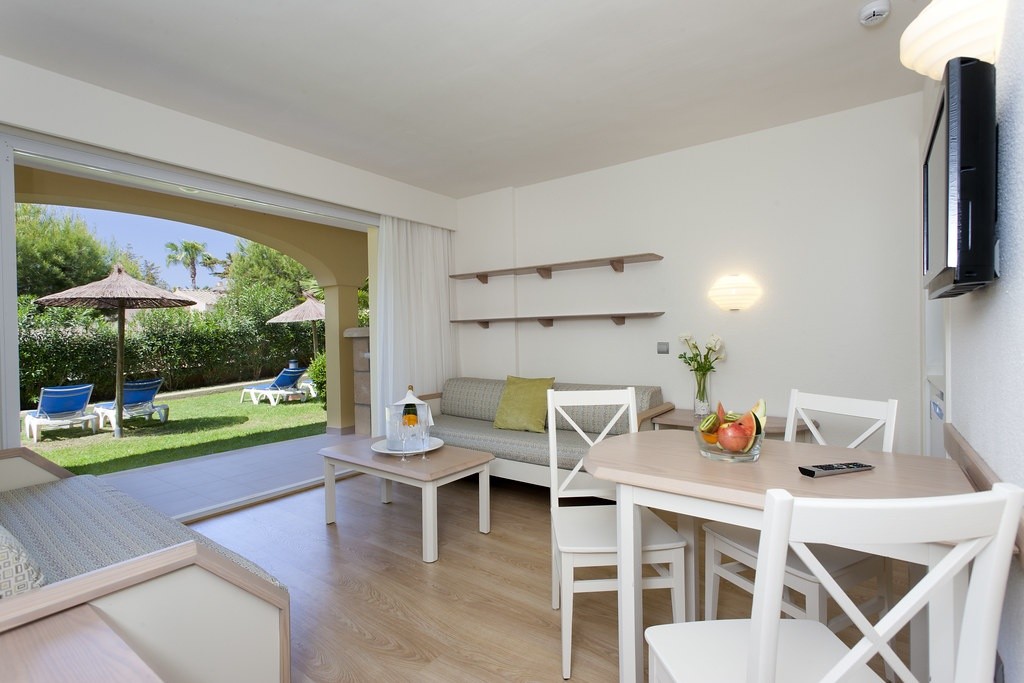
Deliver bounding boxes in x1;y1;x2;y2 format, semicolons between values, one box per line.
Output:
718;421;748;451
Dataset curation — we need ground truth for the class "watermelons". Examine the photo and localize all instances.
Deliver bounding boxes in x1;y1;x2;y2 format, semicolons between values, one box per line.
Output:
734;410;762;453
717;401;725;424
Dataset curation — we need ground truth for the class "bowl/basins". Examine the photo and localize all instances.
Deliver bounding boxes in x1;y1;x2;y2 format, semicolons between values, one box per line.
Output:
693;426;764;463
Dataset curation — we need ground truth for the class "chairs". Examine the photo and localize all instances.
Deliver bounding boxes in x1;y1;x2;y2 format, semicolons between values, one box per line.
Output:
547;387;687;679
92;377;169;430
240;368;307;406
25;384;98;442
645;482;1024;683
701;389;902;683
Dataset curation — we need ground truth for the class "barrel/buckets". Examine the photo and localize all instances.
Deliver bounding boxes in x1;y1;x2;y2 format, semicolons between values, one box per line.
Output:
386;405;431;451
288;360;298;369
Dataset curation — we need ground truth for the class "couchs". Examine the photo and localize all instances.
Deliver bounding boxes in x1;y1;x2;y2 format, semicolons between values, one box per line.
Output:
0;447;291;683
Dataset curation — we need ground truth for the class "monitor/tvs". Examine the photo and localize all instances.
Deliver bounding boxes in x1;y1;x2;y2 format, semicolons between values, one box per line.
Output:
922;57;996;300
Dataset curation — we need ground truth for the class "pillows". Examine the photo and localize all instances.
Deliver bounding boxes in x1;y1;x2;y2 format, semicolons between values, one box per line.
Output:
493;375;555;434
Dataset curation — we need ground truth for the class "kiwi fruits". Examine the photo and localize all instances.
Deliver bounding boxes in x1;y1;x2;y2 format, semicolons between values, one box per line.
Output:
724;413;741;422
699;414;721;434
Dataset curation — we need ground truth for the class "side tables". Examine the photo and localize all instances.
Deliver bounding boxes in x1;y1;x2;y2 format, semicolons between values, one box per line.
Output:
651;408;819;444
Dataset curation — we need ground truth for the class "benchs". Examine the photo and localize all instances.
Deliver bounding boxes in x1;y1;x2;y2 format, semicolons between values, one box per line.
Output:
418;377;664;501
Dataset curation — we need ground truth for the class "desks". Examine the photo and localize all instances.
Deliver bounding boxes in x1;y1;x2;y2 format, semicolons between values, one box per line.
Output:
584;429;1020;683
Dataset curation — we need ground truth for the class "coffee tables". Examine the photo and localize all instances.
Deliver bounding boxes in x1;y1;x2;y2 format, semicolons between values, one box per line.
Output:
319;435;495;563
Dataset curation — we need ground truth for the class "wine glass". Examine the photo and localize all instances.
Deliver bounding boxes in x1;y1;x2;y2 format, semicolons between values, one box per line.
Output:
419;419;429;461
396;419;410;464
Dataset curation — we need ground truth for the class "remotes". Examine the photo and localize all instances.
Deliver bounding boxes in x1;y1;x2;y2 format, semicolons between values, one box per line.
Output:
798;462;876;477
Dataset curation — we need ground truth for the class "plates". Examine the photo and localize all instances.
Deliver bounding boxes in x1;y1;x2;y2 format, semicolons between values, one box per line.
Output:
371;437;444;456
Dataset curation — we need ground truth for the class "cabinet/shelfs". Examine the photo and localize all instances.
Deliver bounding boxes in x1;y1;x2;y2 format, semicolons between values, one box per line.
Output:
449;253;664;325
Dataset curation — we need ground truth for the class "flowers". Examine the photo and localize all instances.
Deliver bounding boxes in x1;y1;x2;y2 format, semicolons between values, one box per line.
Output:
678;332;726;403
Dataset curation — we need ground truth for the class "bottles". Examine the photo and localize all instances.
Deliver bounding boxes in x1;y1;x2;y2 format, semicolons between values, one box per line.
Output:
401;385;418;425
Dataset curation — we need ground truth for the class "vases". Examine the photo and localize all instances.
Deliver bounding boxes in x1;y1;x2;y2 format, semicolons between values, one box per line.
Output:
694;372;711;417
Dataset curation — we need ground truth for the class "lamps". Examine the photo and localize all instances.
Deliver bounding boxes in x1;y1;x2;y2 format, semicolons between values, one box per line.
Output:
899;0;1006;81
710;273;762;312
